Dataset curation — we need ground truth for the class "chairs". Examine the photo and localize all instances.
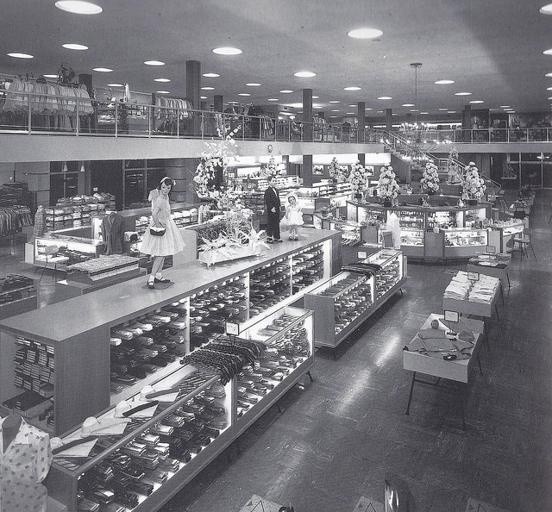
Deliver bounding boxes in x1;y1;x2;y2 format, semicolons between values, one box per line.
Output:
39;245;70;283
512;227;537;261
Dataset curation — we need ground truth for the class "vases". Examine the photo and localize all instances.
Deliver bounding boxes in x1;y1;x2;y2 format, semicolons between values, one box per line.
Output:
427;189;435;195
470;199;477;205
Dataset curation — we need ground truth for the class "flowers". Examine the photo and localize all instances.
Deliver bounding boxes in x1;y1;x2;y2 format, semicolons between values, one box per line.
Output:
421;159;440;192
461;161;486;200
377;166;401;201
329;157;341;179
346;162;369;193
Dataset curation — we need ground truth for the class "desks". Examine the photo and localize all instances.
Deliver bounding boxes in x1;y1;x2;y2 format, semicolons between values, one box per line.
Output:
145;97;193;135
400;250;511;432
5;81;92;131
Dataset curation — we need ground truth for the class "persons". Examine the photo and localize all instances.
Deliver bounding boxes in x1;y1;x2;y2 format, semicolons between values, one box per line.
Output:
0;413;53;512
280;192;305;241
263;175;284;245
99;211;128;256
481;218;489;229
138;175;186;288
474;216;482;229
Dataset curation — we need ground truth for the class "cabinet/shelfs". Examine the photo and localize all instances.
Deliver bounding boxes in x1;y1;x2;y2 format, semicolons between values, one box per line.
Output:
189;270;249;359
40;362;235;512
236;305;317;443
1;325;57;436
109;295;189;408
1;176;405;256
304;268;374;359
292;239;330;303
374;249;403;313
249;252;292;320
0;272;41;321
